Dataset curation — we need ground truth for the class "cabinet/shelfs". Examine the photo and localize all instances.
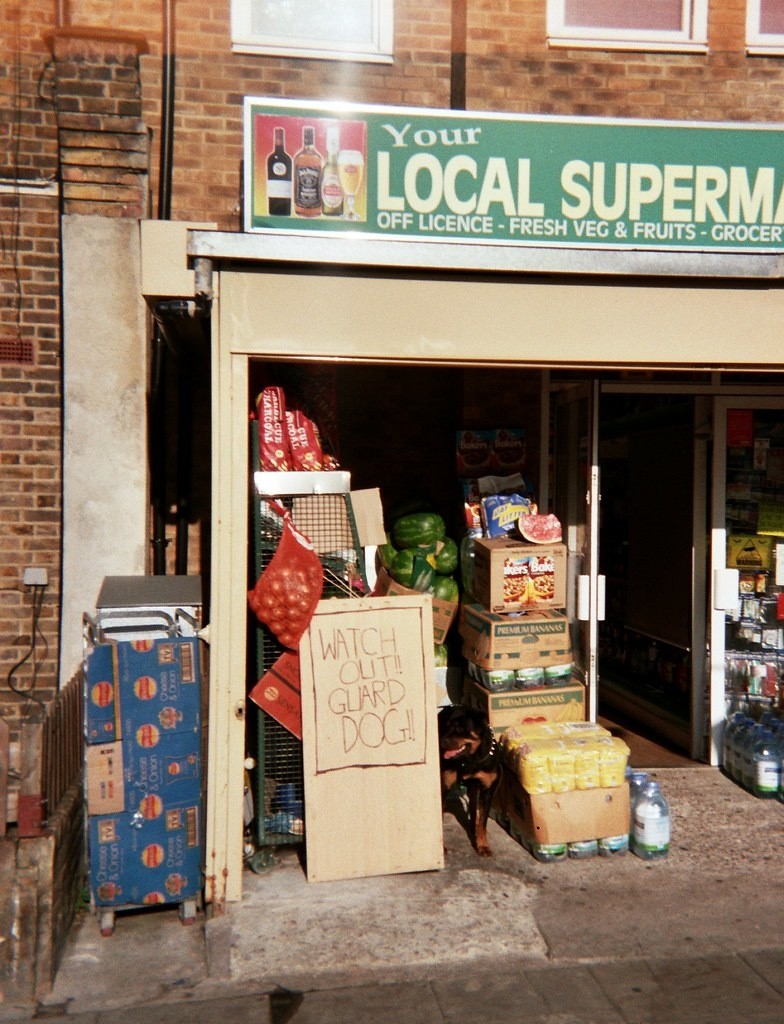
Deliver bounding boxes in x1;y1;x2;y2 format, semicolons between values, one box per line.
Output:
251;418;369;842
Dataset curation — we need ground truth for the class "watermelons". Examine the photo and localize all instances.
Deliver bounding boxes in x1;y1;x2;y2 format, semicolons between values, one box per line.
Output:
518;513;563;544
379;513;460;667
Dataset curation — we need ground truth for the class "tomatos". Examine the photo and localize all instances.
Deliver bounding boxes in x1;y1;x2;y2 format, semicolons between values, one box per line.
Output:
248;541;322;647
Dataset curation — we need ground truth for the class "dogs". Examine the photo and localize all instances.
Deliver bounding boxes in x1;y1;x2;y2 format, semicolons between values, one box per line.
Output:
437;705;504;858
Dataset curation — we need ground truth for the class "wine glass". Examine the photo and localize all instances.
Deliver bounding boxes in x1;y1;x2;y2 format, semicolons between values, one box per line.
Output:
337;150;364;221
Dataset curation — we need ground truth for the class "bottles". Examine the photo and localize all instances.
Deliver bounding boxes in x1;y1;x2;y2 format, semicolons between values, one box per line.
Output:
294;126;324;218
266;126;294;217
723;710;784;802
462;660;575;691
320;128;343;216
484;765;671;865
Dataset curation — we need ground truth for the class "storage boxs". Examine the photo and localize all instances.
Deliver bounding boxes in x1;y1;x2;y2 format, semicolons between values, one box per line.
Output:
470;678;586;744
87;632;204;905
506;783;630;845
375;567;455;647
460;602;575;667
476;537;570;614
